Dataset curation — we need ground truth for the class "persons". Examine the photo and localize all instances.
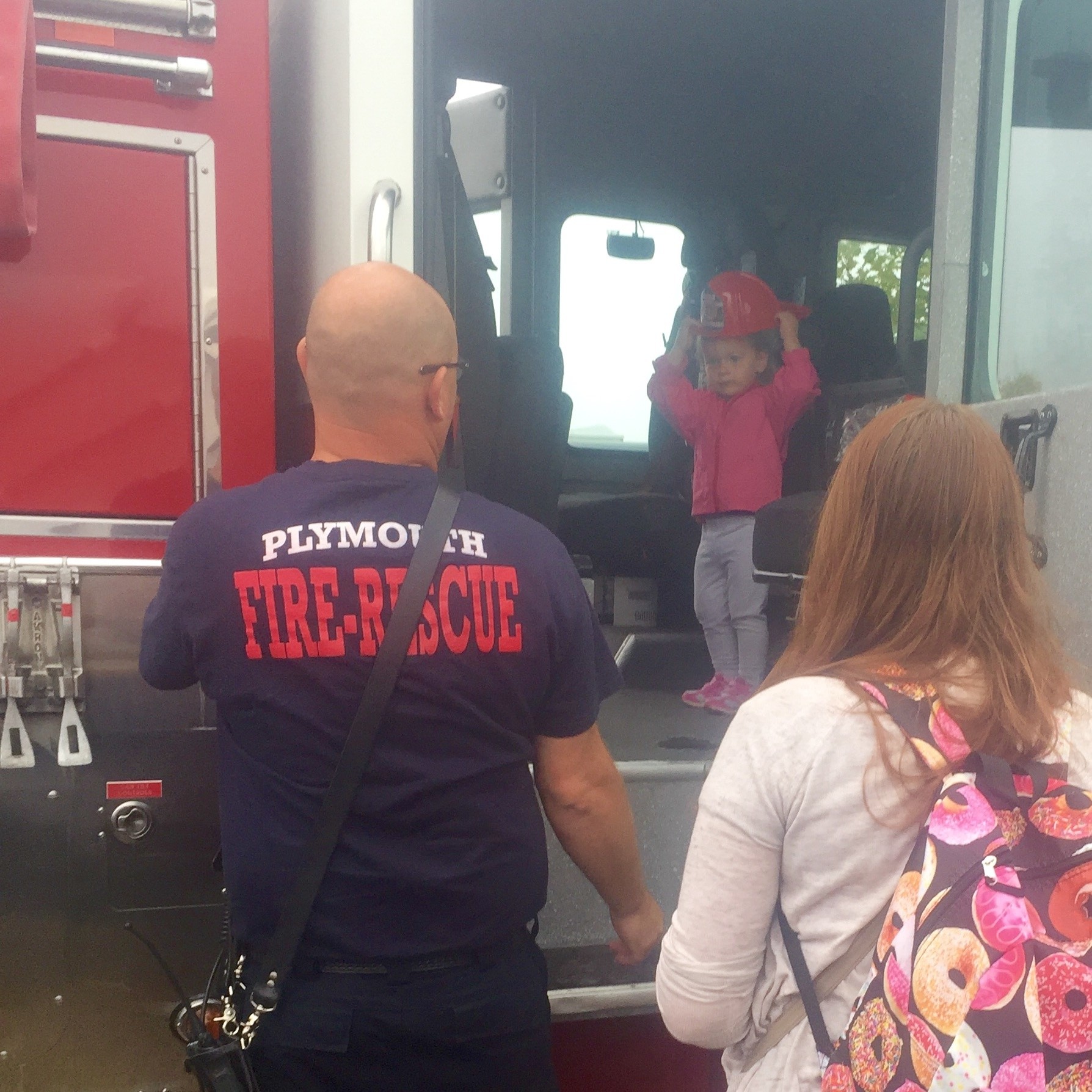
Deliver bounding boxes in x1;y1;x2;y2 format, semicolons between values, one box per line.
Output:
141;256;666;1092
657;396;1092;1091
648;272;823;717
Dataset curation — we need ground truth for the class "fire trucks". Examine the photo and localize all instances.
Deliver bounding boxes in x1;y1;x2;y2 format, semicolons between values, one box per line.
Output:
0;0;1092;1092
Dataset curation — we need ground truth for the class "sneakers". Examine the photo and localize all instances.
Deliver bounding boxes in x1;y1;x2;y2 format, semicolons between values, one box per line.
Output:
706;675;759;716
682;672;729;708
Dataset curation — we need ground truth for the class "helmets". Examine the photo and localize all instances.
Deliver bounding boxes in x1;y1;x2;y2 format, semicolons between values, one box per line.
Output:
691;271;815;335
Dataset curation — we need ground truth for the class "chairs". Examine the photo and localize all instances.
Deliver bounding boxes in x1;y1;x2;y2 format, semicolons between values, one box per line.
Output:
462;276;934;668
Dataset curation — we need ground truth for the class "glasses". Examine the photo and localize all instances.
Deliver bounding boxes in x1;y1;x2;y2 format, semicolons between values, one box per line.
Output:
420;355;471;379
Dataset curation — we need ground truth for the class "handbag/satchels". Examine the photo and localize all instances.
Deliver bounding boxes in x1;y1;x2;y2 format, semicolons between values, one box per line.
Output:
174;989;255;1092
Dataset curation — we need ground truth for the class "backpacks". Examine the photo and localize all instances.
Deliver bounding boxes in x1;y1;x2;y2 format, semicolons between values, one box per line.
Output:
773;660;1092;1091
